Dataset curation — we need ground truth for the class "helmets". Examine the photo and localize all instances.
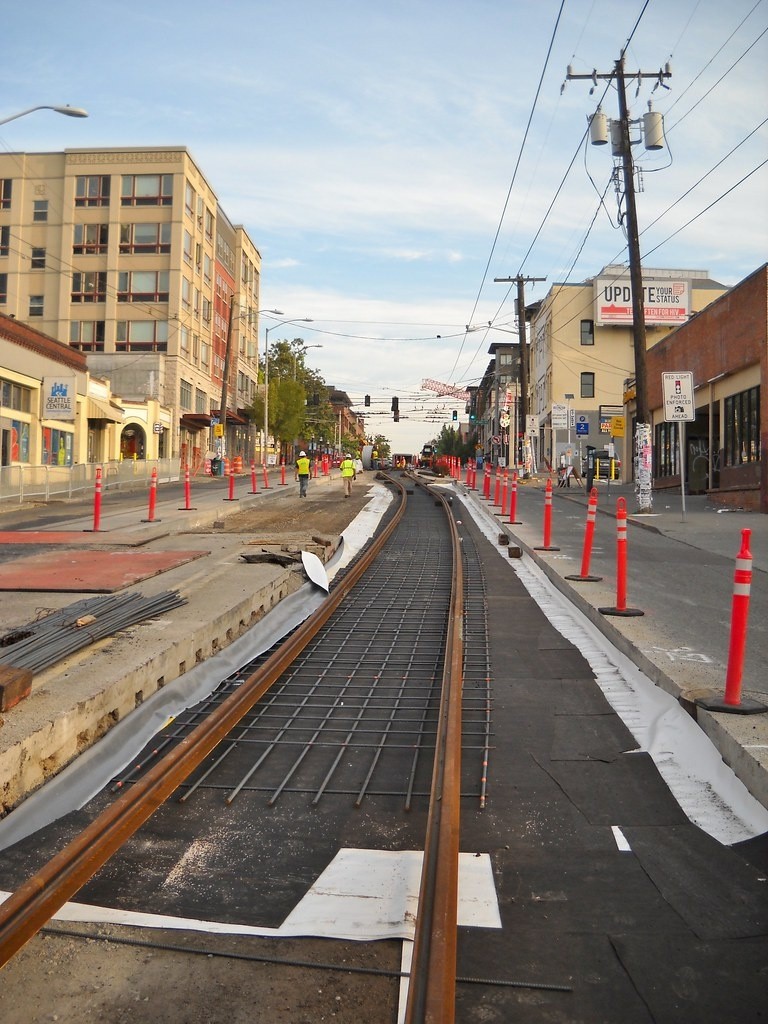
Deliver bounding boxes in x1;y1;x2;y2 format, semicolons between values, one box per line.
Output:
299;451;306;457
346;454;351;458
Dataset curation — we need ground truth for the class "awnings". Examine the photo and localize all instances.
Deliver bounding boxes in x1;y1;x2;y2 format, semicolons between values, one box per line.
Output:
183;409;249;428
87;398;125;424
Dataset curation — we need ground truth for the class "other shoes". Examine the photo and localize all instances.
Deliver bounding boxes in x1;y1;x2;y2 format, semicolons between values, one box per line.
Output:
348;495;350;497
345;495;348;498
302;490;306;497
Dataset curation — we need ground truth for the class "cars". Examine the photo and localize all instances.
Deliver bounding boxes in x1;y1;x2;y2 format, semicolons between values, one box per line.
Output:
582;449;621;481
333;456;347;468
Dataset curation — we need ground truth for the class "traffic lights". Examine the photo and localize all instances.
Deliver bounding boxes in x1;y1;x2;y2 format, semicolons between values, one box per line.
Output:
453;410;458;421
470;409;476;420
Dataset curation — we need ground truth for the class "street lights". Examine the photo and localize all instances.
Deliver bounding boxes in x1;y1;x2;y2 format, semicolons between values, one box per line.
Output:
292;342;325;383
219;309;284;438
263;317;314;466
464;325;532;479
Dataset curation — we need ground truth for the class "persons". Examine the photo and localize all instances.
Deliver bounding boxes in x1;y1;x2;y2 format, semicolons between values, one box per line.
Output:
340;454;356;498
397;461;401;467
418;458;423;467
372;449;378;468
295;451;312;498
485;453;491;465
356;449;361;460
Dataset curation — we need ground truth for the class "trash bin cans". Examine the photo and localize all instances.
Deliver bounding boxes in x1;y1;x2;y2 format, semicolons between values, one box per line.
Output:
212;458;223;477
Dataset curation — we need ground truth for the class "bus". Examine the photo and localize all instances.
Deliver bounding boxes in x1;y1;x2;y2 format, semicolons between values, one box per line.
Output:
419;444;434;465
